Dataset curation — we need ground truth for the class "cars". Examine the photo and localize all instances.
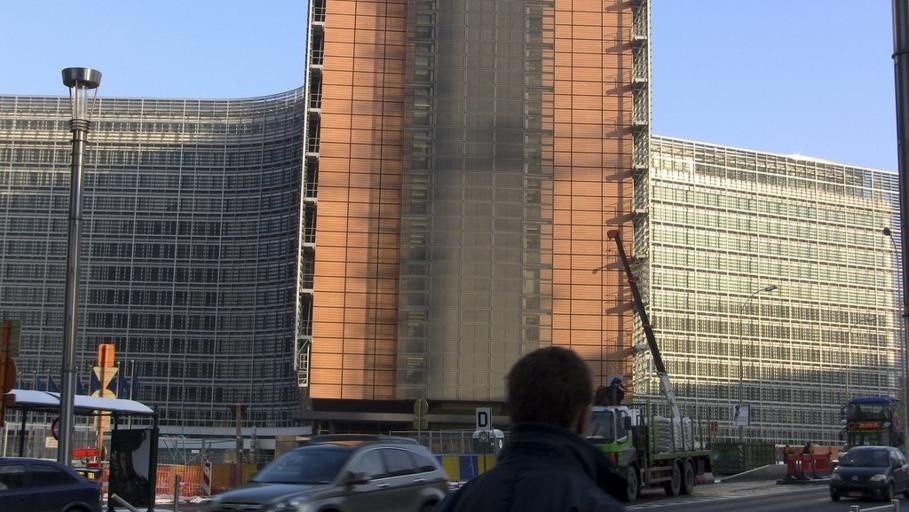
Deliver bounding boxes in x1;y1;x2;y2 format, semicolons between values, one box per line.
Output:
0;456;102;512
827;446;909;506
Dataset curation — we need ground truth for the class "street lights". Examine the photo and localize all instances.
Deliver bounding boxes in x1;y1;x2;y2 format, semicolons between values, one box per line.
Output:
882;226;908;457
845;314;876;404
57;66;103;469
738;284;778;473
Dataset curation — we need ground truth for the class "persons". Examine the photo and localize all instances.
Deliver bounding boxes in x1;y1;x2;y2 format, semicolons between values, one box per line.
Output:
782;440;816;462
827;446;833;459
429;345;630;512
837;449;841;461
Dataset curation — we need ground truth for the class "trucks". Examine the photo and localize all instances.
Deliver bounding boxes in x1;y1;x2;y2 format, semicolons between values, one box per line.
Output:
579;407;715;503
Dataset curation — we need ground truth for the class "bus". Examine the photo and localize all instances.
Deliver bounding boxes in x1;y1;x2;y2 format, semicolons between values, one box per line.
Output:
839;394;908;453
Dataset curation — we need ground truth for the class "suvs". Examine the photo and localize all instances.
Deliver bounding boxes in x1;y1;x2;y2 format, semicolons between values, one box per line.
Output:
197;433;449;511
